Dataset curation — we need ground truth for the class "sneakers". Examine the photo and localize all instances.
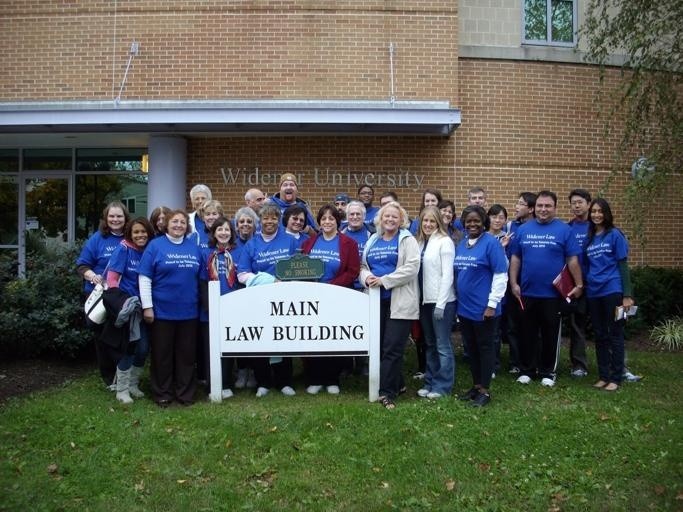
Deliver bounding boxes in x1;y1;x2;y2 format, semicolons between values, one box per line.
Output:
540;378;555;387
413;371;425;380
515;375;531;385
592;380;607;387
326;385;339;394
416;389;441;399
235;368;247;388
306;385;324;395
621;372;644;383
281;386;295;396
459;386;491;407
255;387;270;397
604;383;618;391
570;367;588;377
222;388;233;399
246;367;257;388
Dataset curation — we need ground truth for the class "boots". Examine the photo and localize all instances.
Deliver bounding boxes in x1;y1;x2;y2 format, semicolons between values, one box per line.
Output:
115;365;144;403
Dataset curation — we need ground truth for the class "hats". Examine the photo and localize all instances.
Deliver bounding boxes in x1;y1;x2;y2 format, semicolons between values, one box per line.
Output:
279;172;298;191
334;193;350;204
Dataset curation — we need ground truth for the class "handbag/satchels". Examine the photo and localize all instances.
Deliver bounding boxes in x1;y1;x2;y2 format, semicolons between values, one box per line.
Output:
84;283;106;326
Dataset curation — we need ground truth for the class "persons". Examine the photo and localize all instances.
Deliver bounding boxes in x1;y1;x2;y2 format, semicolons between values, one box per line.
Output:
76;184;644;409
268;173;316;229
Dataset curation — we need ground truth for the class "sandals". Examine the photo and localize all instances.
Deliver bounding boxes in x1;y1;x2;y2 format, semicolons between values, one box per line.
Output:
379;395;396;410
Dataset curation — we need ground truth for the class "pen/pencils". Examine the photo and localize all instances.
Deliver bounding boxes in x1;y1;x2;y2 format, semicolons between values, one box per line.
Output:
623;312;627;320
519;297;525;311
